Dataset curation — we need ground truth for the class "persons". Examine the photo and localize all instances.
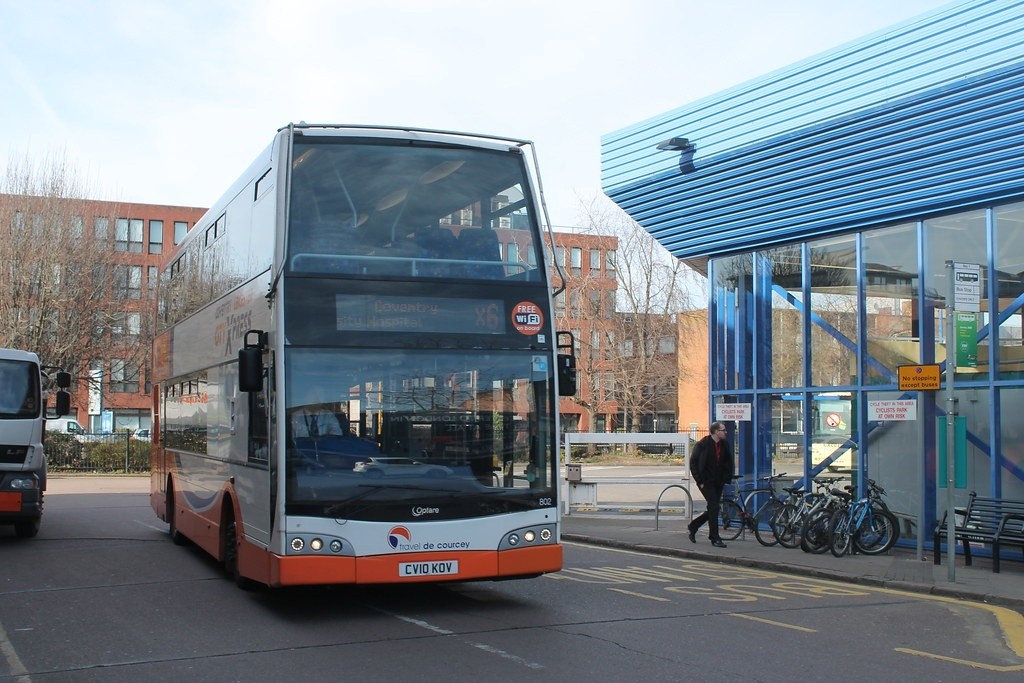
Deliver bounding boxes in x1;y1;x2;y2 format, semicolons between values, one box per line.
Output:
67;423;72;431
291;385;343;438
687;421;734;547
333;400;348;431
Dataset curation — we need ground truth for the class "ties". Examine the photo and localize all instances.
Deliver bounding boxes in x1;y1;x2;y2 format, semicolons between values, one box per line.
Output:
310;414;319;436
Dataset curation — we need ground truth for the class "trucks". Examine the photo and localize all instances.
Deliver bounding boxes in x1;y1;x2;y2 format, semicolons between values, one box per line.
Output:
44;418;101;444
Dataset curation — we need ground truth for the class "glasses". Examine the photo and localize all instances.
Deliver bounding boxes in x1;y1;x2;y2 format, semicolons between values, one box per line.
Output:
718;429;727;432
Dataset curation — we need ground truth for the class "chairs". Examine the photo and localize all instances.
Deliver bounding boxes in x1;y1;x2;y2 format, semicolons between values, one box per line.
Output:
416;227;455;248
458;228;505;279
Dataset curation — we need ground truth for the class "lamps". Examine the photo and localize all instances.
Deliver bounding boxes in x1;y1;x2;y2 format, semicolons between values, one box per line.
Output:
655;137;696;151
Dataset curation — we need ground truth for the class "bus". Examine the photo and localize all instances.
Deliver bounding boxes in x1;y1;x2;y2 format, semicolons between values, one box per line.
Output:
0;346;71;538
149;120;578;595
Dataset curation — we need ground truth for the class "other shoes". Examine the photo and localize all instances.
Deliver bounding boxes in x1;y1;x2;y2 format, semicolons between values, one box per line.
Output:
688;523;698;543
712;537;727;547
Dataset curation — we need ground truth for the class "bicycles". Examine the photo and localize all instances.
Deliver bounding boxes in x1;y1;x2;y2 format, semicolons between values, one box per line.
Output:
718;471;900;558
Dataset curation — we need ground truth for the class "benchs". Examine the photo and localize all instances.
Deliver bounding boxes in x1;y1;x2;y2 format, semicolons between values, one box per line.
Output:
934;491;1024;574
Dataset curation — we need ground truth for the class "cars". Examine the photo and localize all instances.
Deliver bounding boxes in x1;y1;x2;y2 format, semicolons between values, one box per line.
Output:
129;429;151;443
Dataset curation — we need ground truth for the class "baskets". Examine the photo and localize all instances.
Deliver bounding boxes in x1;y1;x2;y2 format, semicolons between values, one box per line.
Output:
773;477;792;498
